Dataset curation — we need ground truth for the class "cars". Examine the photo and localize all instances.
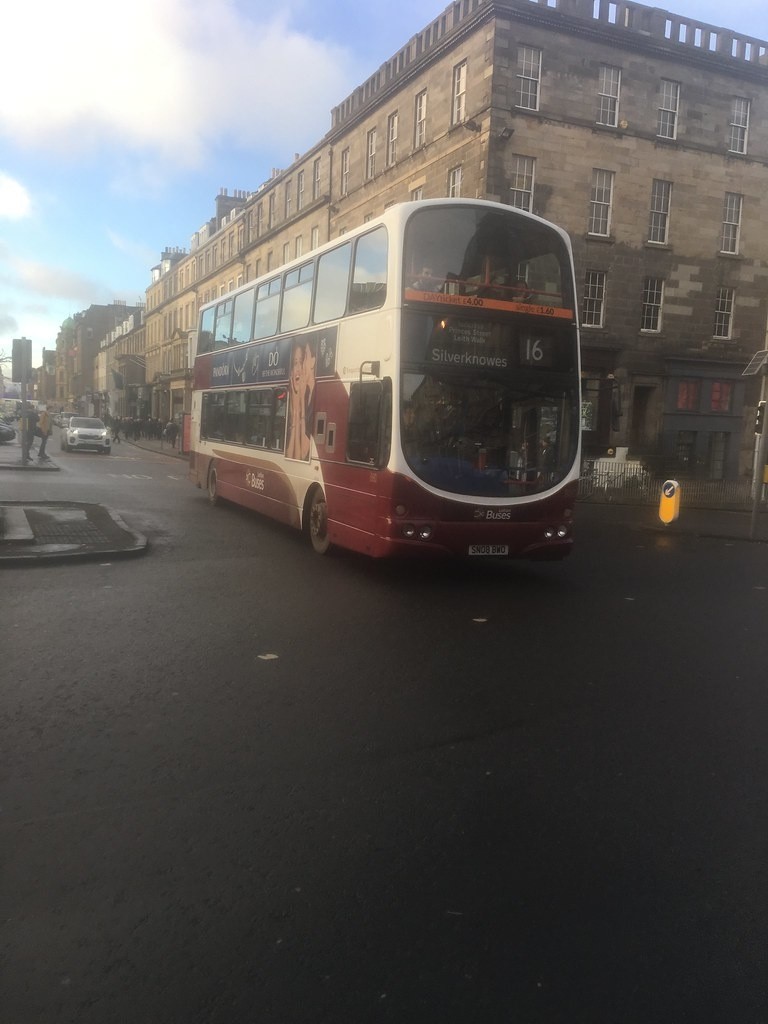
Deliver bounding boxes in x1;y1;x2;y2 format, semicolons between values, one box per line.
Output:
51;413;75;428
59;416;112;456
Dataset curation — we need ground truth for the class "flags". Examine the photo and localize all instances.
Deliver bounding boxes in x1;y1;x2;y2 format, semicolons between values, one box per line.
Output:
111;368;125;390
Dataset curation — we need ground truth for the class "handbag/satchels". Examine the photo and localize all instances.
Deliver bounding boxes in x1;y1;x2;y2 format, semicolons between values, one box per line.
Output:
35;426;42;437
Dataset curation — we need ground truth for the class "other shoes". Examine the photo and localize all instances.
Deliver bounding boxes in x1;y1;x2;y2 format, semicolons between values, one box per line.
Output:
38;453;49;459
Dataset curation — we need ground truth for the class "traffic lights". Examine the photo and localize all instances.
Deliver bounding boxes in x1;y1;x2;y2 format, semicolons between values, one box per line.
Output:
754;400;766;436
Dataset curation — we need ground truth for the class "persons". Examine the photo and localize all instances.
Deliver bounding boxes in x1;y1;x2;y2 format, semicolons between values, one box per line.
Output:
485;272;538;304
22;404;53;462
401;404;422;443
285;338;316;461
91;412;182;448
412;264;441;292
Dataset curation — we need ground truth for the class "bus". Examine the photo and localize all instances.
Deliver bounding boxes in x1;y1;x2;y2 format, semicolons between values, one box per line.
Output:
178;197;626;572
